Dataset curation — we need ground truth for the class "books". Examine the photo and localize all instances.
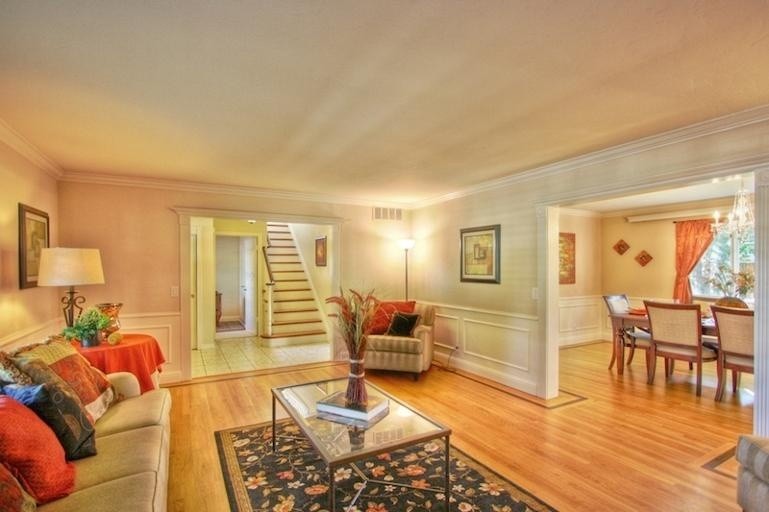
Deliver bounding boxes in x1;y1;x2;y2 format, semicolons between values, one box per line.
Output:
317;390;389;421
316;407;389;430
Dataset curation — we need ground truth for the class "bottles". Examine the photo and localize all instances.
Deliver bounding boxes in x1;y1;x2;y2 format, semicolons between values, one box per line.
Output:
96;301;123;344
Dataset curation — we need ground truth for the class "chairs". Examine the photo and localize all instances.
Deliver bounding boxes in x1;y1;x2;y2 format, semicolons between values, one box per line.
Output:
733;435;769;511
603;294;755;402
364;305;434;380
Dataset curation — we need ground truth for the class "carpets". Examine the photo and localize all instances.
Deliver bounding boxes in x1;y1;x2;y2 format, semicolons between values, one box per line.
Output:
216;320;246;331
214;417;559;511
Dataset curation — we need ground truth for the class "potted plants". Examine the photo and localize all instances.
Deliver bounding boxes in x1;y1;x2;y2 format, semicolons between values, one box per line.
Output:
59;307;110;347
325;287;397;402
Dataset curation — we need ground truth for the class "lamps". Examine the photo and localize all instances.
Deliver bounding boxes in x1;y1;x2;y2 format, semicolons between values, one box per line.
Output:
399;240;413;301
38;247;105;327
711;178;754;239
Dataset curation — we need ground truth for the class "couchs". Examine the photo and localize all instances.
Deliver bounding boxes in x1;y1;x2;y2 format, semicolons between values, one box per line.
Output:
37;372;172;512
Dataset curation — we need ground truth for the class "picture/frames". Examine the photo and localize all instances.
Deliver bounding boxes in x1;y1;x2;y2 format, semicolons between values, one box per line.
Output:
460;224;500;283
315;236;327;266
18;203;49;289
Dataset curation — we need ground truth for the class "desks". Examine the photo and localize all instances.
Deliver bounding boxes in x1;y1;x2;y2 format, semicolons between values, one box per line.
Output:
70;334;165;394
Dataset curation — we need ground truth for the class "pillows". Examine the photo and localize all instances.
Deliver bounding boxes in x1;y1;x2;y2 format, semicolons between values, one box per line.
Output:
384;311;421;337
363;302;415;334
0;335;124;512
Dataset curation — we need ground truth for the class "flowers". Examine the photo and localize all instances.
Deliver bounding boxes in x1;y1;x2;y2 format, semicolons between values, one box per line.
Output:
706;266;755;299
